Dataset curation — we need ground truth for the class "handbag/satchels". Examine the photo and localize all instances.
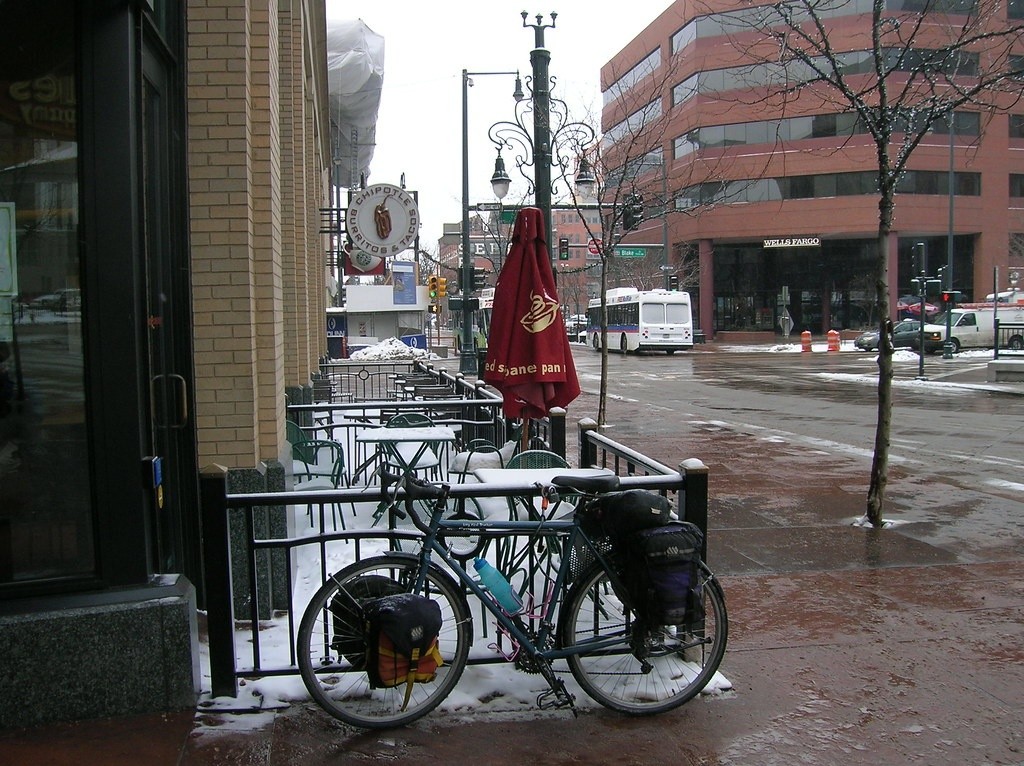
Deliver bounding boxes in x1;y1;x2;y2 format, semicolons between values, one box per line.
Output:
577;493;673;537
614;517;705;670
358;594;443;715
327;575;408;674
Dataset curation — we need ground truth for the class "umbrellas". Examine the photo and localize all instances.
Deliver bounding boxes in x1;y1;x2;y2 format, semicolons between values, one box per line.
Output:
484;206;581;469
908;302;940;315
897;301;908;311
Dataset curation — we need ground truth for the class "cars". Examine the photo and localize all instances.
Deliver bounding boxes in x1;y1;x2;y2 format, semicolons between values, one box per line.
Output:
853;319;929;352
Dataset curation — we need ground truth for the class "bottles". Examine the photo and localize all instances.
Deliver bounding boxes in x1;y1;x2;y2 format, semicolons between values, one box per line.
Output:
473;557;523;614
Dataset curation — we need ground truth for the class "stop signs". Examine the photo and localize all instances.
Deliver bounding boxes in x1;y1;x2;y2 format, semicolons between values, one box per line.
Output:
585;237;606;260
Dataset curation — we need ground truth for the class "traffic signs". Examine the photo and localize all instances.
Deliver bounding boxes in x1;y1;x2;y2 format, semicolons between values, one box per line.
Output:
477;203;504;213
659;265;676;271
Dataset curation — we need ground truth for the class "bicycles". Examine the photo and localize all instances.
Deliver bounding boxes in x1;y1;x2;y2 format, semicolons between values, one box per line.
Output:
294;464;731;732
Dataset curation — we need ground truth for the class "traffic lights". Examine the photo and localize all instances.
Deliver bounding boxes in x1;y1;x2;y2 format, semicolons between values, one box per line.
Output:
469;298;480;311
429;304;438;314
472;265;489;290
428;275;439;299
668;274;679;292
437;277;450;298
621;193;645;230
942;292;955;304
440;305;443;313
558;237;569;262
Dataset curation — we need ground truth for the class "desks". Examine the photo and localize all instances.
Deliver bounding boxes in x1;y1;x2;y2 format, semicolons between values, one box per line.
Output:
402;386;414;401
474;469;608;487
394;379;406;399
356;428;456;528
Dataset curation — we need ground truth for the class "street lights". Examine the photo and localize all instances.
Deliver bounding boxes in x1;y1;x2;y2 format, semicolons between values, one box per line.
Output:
924;106;956;358
459;68;523;378
488;8;595;289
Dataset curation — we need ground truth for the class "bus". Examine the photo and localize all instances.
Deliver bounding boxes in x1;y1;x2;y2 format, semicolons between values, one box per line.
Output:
584;285;695;356
451;288;499;355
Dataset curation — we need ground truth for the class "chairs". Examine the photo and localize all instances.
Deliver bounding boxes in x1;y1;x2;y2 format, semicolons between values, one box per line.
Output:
286;420;355;528
447;424;543;520
493;450;572;522
384;413;440;486
386;371;463;426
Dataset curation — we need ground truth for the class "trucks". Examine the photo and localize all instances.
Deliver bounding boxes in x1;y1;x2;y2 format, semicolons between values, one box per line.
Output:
984;291;1024;306
917;307;1024;354
